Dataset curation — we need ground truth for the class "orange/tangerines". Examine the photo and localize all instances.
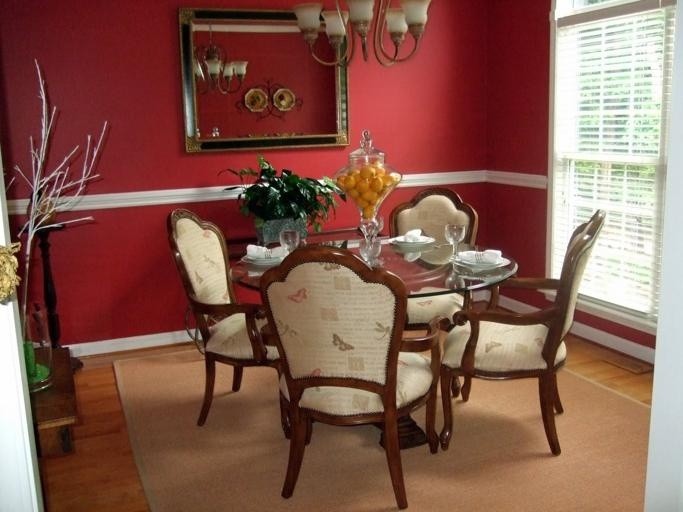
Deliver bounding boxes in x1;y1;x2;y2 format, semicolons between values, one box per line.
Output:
336;161;399;219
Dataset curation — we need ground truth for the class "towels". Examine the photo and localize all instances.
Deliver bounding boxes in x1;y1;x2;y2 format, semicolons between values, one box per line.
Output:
456;248;502;266
401;251;421;263
245;244;287;259
402;228;423;243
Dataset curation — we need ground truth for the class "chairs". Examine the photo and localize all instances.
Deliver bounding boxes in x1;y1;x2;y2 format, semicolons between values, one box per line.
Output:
257;242;444;509
164;206;297;440
436;207;607;460
382;185;480;404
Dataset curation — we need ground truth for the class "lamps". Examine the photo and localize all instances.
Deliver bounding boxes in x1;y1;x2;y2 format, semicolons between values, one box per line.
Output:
291;0;440;70
190;20;249;97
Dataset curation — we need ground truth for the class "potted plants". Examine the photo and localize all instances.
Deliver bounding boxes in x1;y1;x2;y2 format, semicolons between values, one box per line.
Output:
212;148;348;251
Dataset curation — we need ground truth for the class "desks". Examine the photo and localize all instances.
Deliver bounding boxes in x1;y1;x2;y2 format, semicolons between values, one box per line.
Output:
22;345;79;432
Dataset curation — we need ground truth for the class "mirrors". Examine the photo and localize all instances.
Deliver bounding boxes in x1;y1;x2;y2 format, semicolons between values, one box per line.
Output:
175;5;354;159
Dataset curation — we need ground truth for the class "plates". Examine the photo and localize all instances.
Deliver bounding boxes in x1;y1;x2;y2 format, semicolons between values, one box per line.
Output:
238;252;282;267
456;270;506;281
454;250;511;270
392;246;434;254
386;235;436;246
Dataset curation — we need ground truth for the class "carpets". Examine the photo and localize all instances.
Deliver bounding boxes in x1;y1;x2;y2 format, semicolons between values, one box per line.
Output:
109;329;652;511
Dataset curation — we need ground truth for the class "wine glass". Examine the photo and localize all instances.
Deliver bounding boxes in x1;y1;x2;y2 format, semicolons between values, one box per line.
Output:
443;223;466;261
279;230;297;255
443;262;465;290
